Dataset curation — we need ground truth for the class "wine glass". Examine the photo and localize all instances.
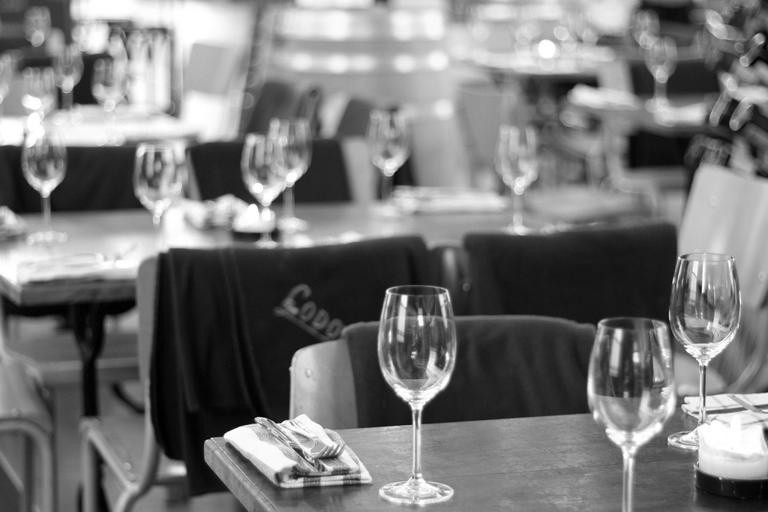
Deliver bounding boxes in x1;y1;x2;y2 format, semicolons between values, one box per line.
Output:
20;115;69;244
366;109;413;235
492;122;542;236
133;143;183;256
588;254;742;512
241;114;315;247
377;284;457;505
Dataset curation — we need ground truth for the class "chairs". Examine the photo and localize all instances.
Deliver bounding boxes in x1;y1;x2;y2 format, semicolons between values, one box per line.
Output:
288;315;602;434
76;231;435;510
0;324;61;510
457;220;679;321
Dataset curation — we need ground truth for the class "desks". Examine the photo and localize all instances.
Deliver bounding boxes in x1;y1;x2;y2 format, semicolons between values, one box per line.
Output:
1;49;722;188
0;187;649;421
